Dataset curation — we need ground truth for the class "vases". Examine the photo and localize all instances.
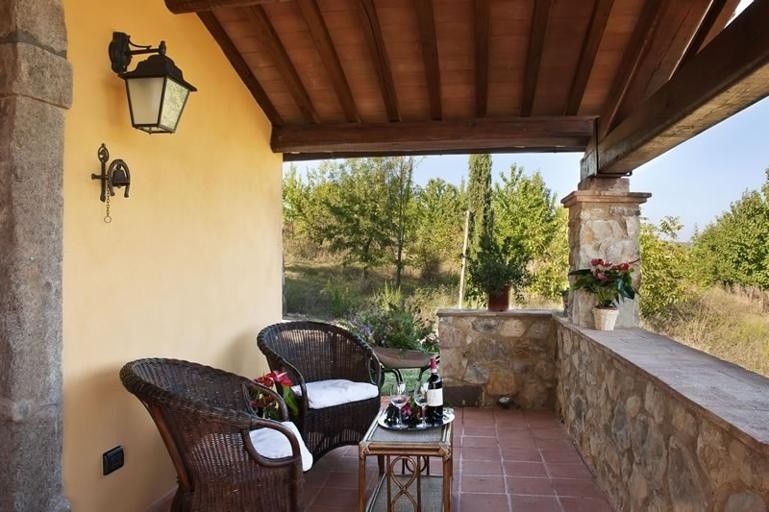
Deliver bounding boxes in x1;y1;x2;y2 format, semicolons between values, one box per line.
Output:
591;303;620;332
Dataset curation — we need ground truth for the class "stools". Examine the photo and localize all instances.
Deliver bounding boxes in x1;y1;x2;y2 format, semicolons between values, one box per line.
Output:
372;345;440;386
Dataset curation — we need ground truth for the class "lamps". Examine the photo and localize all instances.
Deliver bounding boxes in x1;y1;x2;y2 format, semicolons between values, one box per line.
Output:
106;27;199;138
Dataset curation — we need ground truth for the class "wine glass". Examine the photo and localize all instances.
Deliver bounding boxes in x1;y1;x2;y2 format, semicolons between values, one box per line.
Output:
389;382;407;430
413;382;432;431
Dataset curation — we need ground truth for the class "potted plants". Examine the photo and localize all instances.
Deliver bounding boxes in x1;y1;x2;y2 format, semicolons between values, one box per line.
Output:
455;204;554;312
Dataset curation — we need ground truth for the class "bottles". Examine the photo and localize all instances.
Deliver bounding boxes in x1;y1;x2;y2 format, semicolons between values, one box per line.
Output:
423;354;445;425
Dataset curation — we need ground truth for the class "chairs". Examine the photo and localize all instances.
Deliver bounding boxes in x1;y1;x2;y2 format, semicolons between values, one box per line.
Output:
257;319;388;479
118;356;309;512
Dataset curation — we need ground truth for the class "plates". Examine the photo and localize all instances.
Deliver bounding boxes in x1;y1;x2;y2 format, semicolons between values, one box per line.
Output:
377;409;456;431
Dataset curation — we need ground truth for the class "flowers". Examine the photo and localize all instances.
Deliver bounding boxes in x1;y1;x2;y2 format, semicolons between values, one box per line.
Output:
566;255;641;307
246;367;299;423
